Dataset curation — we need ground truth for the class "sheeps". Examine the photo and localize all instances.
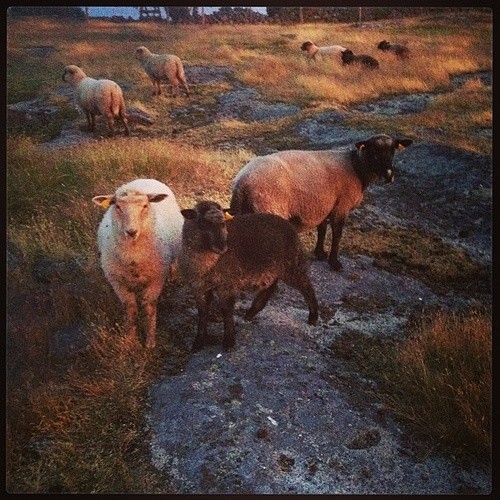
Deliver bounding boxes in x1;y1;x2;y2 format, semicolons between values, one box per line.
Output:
377;40;411;62
340;49;380;70
92;178;185;349
62;64;129;138
231;134;409;273
300;41;347;63
181;201;319;351
133;46;190;98
